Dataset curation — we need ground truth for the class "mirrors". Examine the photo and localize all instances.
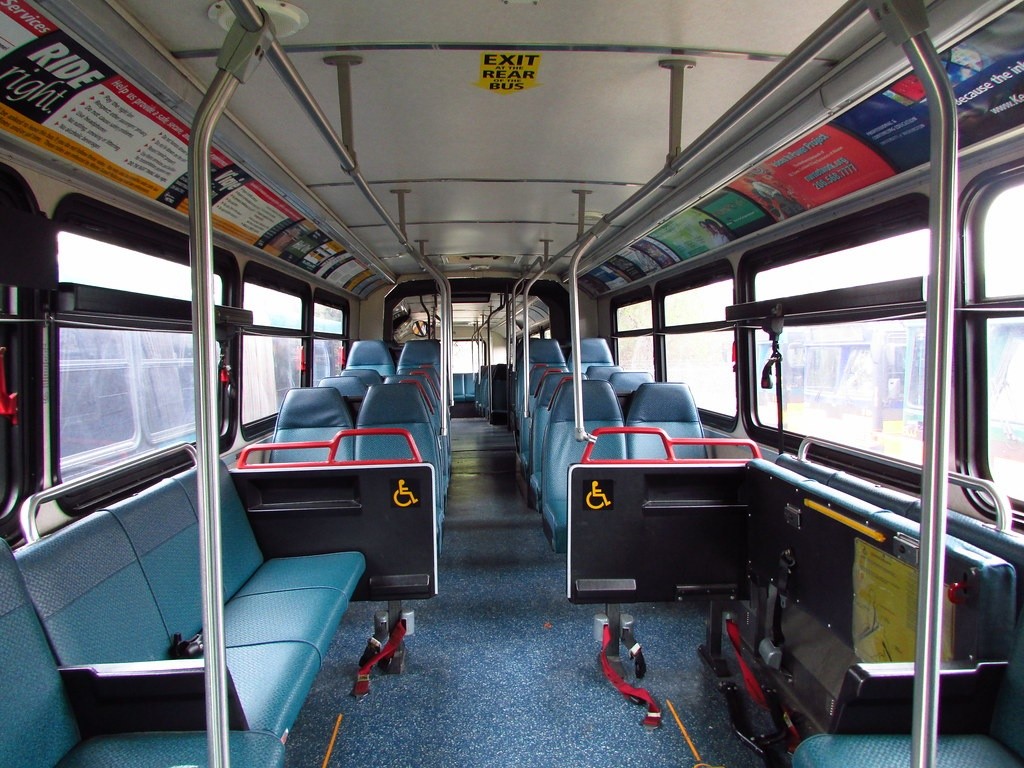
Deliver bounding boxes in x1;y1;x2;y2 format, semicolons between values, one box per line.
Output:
412;320;428;337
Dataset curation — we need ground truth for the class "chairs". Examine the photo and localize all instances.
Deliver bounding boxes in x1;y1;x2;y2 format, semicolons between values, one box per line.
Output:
1;335;1024;767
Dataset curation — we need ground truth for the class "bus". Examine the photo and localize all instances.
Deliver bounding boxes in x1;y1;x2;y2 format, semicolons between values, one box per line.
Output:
0;1;1023;768
